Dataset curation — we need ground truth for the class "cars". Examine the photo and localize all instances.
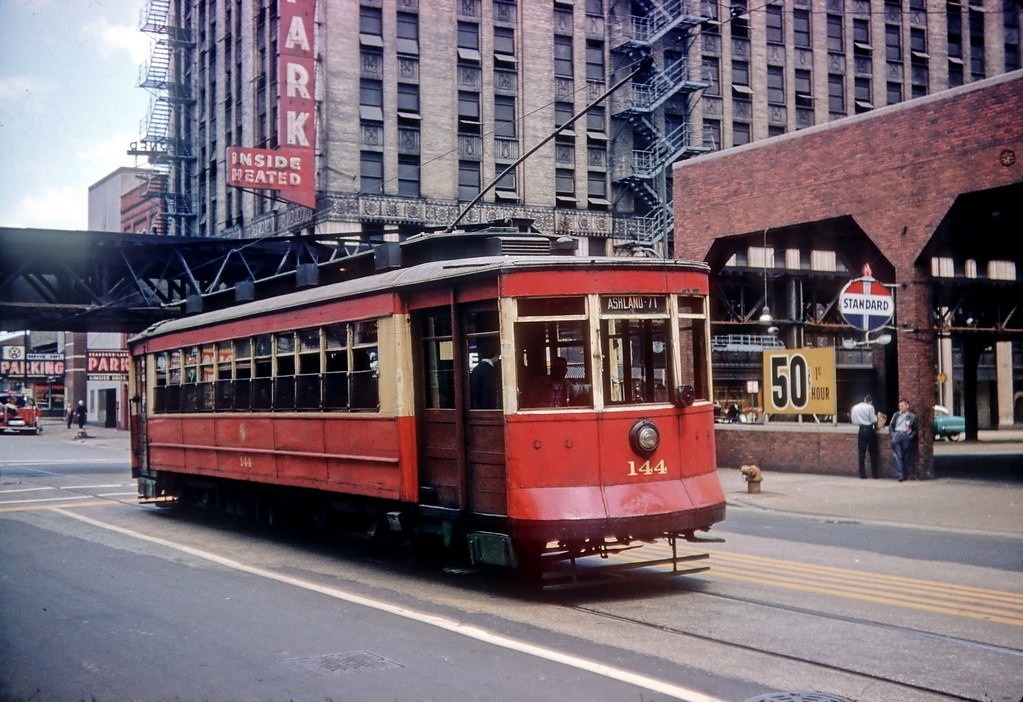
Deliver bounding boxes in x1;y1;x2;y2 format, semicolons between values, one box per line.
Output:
0;393;42;434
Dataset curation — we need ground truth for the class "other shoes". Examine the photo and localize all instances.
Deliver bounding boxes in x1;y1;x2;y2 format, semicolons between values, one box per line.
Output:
872;473;878;478
898;477;907;482
860;474;868;478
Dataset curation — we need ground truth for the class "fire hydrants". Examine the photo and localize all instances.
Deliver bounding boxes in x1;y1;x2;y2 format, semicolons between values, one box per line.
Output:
741;464;762;494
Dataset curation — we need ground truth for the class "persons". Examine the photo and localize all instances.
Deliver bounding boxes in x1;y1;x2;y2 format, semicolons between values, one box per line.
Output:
470;338;500;409
8;396;22;407
889;398;918;482
77;400;88;431
850;392;879;479
64;402;75;429
536;357;573;407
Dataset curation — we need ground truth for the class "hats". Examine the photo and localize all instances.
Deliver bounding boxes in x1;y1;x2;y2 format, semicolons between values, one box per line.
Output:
79;400;85;404
479;337;498;358
552;357;567;367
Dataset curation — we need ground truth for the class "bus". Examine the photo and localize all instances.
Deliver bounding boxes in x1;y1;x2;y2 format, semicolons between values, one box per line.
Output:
126;52;726;592
